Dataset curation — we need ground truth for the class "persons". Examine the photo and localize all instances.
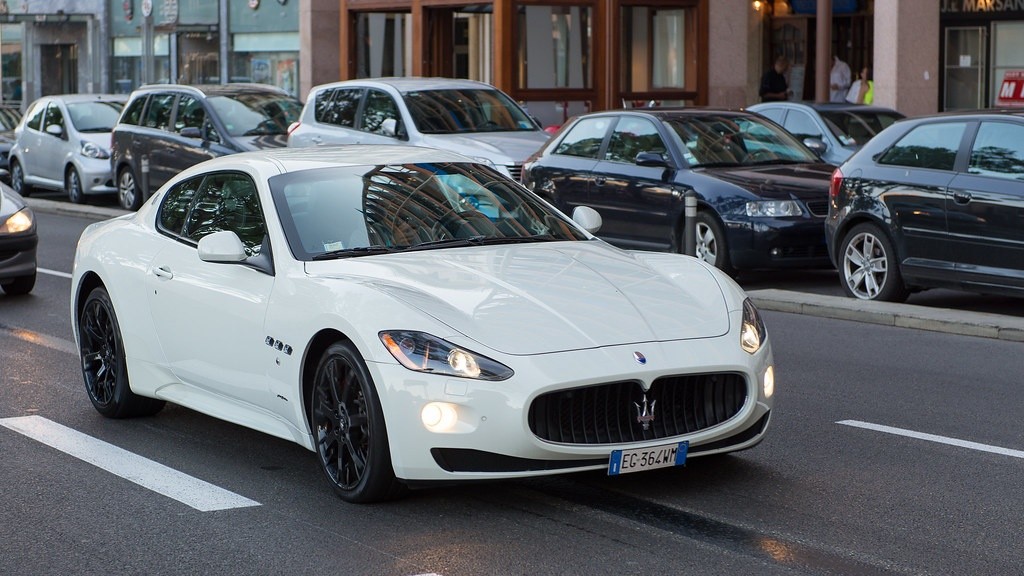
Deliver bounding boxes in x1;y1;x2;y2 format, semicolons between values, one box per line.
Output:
829;51;852;104
846;66;874;106
758;57;794;102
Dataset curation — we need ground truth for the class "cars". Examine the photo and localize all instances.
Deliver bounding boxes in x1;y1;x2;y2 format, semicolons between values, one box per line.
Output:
825;113;1024;302
746;101;907;168
7;94;127;201
520;107;836;277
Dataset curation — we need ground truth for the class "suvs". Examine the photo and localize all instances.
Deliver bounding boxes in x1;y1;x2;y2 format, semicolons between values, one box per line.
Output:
288;76;553;184
111;81;305;208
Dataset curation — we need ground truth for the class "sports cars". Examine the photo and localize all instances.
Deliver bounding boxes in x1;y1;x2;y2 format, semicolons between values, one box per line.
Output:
75;144;774;506
